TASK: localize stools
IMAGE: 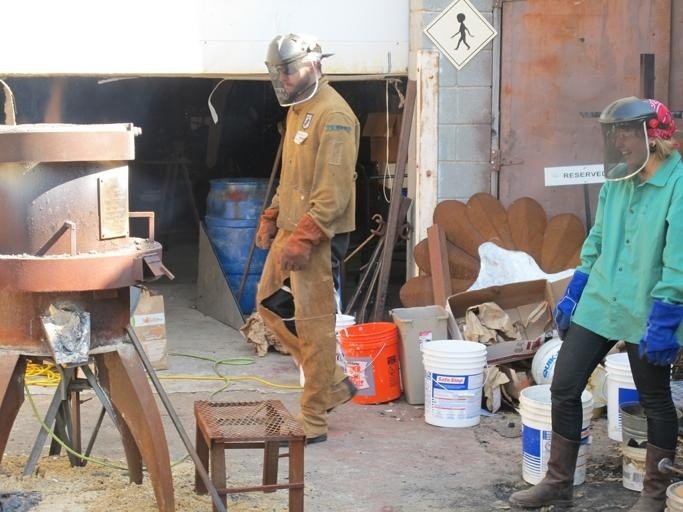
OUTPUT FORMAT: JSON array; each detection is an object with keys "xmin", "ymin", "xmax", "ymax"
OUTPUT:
[{"xmin": 193, "ymin": 399, "xmax": 306, "ymax": 512}]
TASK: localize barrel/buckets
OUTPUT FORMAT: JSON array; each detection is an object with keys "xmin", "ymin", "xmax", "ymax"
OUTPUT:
[
  {"xmin": 420, "ymin": 339, "xmax": 488, "ymax": 428},
  {"xmin": 299, "ymin": 314, "xmax": 357, "ymax": 389},
  {"xmin": 531, "ymin": 338, "xmax": 607, "ymax": 409},
  {"xmin": 206, "ymin": 179, "xmax": 270, "ymax": 314},
  {"xmin": 664, "ymin": 481, "xmax": 683, "ymax": 512},
  {"xmin": 339, "ymin": 321, "xmax": 400, "ymax": 404},
  {"xmin": 621, "ymin": 403, "xmax": 682, "ymax": 492},
  {"xmin": 604, "ymin": 352, "xmax": 641, "ymax": 440},
  {"xmin": 519, "ymin": 384, "xmax": 594, "ymax": 487}
]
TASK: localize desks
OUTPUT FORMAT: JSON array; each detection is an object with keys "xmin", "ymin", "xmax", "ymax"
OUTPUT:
[{"xmin": 136, "ymin": 159, "xmax": 201, "ymax": 226}]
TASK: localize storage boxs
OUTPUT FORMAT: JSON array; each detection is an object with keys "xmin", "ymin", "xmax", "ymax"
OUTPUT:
[
  {"xmin": 362, "ymin": 112, "xmax": 404, "ymax": 163},
  {"xmin": 129, "ymin": 285, "xmax": 167, "ymax": 370},
  {"xmin": 443, "ymin": 278, "xmax": 559, "ymax": 366}
]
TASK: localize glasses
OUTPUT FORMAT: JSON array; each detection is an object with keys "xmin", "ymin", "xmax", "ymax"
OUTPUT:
[
  {"xmin": 269, "ymin": 64, "xmax": 303, "ymax": 80},
  {"xmin": 606, "ymin": 129, "xmax": 635, "ymax": 144}
]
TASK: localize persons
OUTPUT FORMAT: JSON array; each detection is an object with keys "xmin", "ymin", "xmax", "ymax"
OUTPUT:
[
  {"xmin": 508, "ymin": 98, "xmax": 682, "ymax": 512},
  {"xmin": 254, "ymin": 33, "xmax": 363, "ymax": 447}
]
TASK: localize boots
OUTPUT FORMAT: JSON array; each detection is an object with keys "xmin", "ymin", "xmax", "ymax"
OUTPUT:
[
  {"xmin": 510, "ymin": 431, "xmax": 581, "ymax": 509},
  {"xmin": 628, "ymin": 442, "xmax": 676, "ymax": 512}
]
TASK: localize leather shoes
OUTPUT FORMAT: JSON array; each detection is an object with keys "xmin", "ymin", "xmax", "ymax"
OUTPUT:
[
  {"xmin": 328, "ymin": 379, "xmax": 357, "ymax": 413},
  {"xmin": 280, "ymin": 433, "xmax": 328, "ymax": 448}
]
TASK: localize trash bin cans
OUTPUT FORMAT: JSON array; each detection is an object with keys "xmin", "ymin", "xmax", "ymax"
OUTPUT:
[{"xmin": 389, "ymin": 304, "xmax": 449, "ymax": 405}]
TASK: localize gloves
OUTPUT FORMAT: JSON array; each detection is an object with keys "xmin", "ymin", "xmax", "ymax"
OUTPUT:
[
  {"xmin": 276, "ymin": 213, "xmax": 329, "ymax": 272},
  {"xmin": 553, "ymin": 270, "xmax": 590, "ymax": 341},
  {"xmin": 638, "ymin": 299, "xmax": 683, "ymax": 366},
  {"xmin": 254, "ymin": 209, "xmax": 277, "ymax": 250}
]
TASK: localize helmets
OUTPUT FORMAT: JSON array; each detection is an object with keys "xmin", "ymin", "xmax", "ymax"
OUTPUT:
[
  {"xmin": 599, "ymin": 96, "xmax": 676, "ymax": 139},
  {"xmin": 264, "ymin": 33, "xmax": 322, "ymax": 68}
]
